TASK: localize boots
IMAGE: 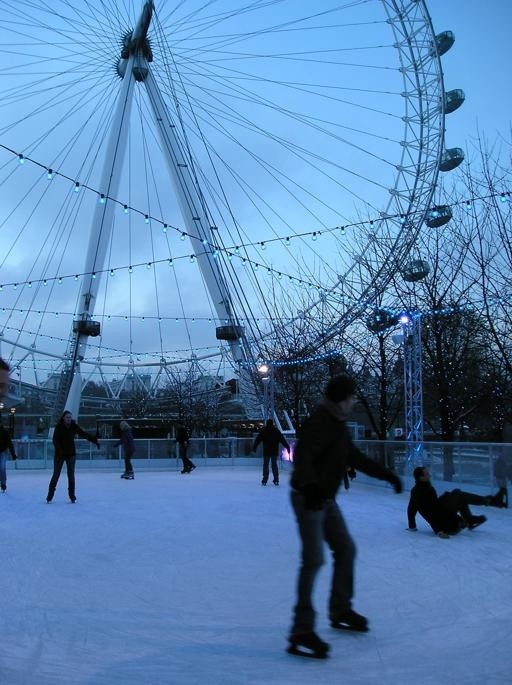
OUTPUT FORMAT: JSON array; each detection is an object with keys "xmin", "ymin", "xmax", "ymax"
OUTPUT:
[
  {"xmin": 287, "ymin": 619, "xmax": 332, "ymax": 653},
  {"xmin": 463, "ymin": 486, "xmax": 506, "ymax": 527},
  {"xmin": 329, "ymin": 601, "xmax": 370, "ymax": 627}
]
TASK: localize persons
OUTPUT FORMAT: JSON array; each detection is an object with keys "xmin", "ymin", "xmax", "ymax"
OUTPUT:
[
  {"xmin": 0, "ymin": 424, "xmax": 15, "ymax": 494},
  {"xmin": 344, "ymin": 468, "xmax": 358, "ymax": 491},
  {"xmin": 404, "ymin": 465, "xmax": 508, "ymax": 539},
  {"xmin": 111, "ymin": 420, "xmax": 136, "ymax": 479},
  {"xmin": 284, "ymin": 375, "xmax": 402, "ymax": 661},
  {"xmin": 252, "ymin": 418, "xmax": 291, "ymax": 486},
  {"xmin": 173, "ymin": 421, "xmax": 196, "ymax": 473},
  {"xmin": 44, "ymin": 409, "xmax": 99, "ymax": 504},
  {"xmin": 0, "ymin": 356, "xmax": 12, "ymax": 409}
]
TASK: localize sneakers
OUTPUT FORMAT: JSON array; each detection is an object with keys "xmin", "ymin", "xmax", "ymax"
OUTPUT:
[{"xmin": 1, "ymin": 461, "xmax": 279, "ymax": 501}]
